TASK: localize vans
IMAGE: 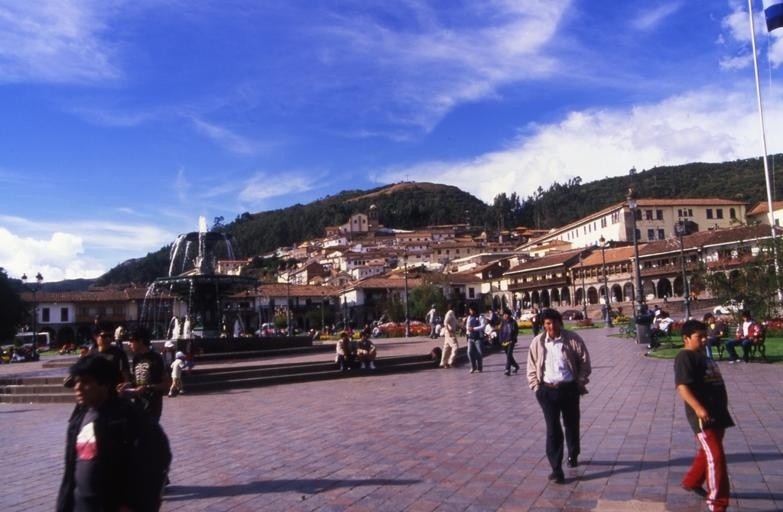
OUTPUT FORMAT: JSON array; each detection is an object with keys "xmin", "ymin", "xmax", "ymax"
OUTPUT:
[{"xmin": 254, "ymin": 323, "xmax": 287, "ymax": 338}]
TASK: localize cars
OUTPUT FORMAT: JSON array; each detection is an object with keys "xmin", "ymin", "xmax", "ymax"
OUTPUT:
[
  {"xmin": 761, "ymin": 314, "xmax": 782, "ymax": 330},
  {"xmin": 712, "ymin": 296, "xmax": 744, "ymax": 316},
  {"xmin": 560, "ymin": 310, "xmax": 583, "ymax": 322},
  {"xmin": 318, "ymin": 327, "xmax": 361, "ymax": 342},
  {"xmin": 371, "ymin": 318, "xmax": 429, "ymax": 339},
  {"xmin": 599, "ymin": 310, "xmax": 625, "ymax": 320},
  {"xmin": 518, "ymin": 314, "xmax": 538, "ymax": 328},
  {"xmin": 57, "ymin": 338, "xmax": 96, "ymax": 354}
]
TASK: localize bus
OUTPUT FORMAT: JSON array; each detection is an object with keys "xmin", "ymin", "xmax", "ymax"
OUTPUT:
[{"xmin": 12, "ymin": 332, "xmax": 50, "ymax": 353}]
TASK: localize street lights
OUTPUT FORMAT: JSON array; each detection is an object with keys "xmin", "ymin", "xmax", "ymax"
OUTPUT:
[
  {"xmin": 625, "ymin": 183, "xmax": 647, "ymax": 317},
  {"xmin": 598, "ymin": 233, "xmax": 612, "ymax": 326},
  {"xmin": 244, "ymin": 288, "xmax": 262, "ymax": 337},
  {"xmin": 21, "ymin": 271, "xmax": 43, "ymax": 359},
  {"xmin": 319, "ymin": 291, "xmax": 325, "ymax": 333},
  {"xmin": 487, "ymin": 269, "xmax": 494, "ymax": 312},
  {"xmin": 577, "ymin": 253, "xmax": 588, "ymax": 320},
  {"xmin": 341, "ymin": 284, "xmax": 349, "ymax": 332},
  {"xmin": 673, "ymin": 217, "xmax": 693, "ymax": 322},
  {"xmin": 402, "ymin": 263, "xmax": 409, "ymax": 337}
]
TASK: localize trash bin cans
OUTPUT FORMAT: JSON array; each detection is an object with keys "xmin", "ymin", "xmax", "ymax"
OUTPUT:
[{"xmin": 635, "ymin": 315, "xmax": 651, "ymax": 344}]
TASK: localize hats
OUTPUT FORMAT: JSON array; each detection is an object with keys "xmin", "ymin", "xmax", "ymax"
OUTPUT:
[{"xmin": 176, "ymin": 351, "xmax": 185, "ymax": 358}]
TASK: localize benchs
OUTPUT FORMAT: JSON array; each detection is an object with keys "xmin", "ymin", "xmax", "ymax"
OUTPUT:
[
  {"xmin": 710, "ymin": 325, "xmax": 768, "ymax": 362},
  {"xmin": 652, "ymin": 323, "xmax": 675, "ymax": 351}
]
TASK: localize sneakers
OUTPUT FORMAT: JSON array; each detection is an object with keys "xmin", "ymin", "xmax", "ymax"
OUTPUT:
[
  {"xmin": 504, "ymin": 369, "xmax": 510, "ymax": 376},
  {"xmin": 513, "ymin": 365, "xmax": 520, "ymax": 373}
]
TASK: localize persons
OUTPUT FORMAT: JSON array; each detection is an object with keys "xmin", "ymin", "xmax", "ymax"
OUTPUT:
[
  {"xmin": 333, "ymin": 332, "xmax": 353, "ymax": 372},
  {"xmin": 327, "ymin": 322, "xmax": 336, "ymax": 335},
  {"xmin": 645, "ymin": 304, "xmax": 673, "ymax": 348},
  {"xmin": 372, "ymin": 323, "xmax": 382, "ymax": 337},
  {"xmin": 0, "ymin": 345, "xmax": 40, "ymax": 364},
  {"xmin": 725, "ymin": 309, "xmax": 762, "ymax": 363},
  {"xmin": 53, "ymin": 322, "xmax": 193, "ymax": 512},
  {"xmin": 423, "ymin": 300, "xmax": 521, "ymax": 376},
  {"xmin": 354, "ymin": 332, "xmax": 376, "ymax": 369},
  {"xmin": 701, "ymin": 313, "xmax": 726, "ymax": 360},
  {"xmin": 525, "ymin": 308, "xmax": 591, "ymax": 482},
  {"xmin": 672, "ymin": 319, "xmax": 736, "ymax": 511},
  {"xmin": 528, "ymin": 308, "xmax": 540, "ymax": 336},
  {"xmin": 361, "ymin": 323, "xmax": 371, "ymax": 338}
]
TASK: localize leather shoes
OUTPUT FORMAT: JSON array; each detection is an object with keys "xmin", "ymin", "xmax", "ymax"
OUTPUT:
[
  {"xmin": 682, "ymin": 484, "xmax": 707, "ymax": 498},
  {"xmin": 548, "ymin": 473, "xmax": 564, "ymax": 484},
  {"xmin": 569, "ymin": 457, "xmax": 577, "ymax": 467}
]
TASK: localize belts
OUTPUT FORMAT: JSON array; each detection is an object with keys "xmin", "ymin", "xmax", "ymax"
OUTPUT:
[{"xmin": 543, "ymin": 382, "xmax": 561, "ymax": 388}]
